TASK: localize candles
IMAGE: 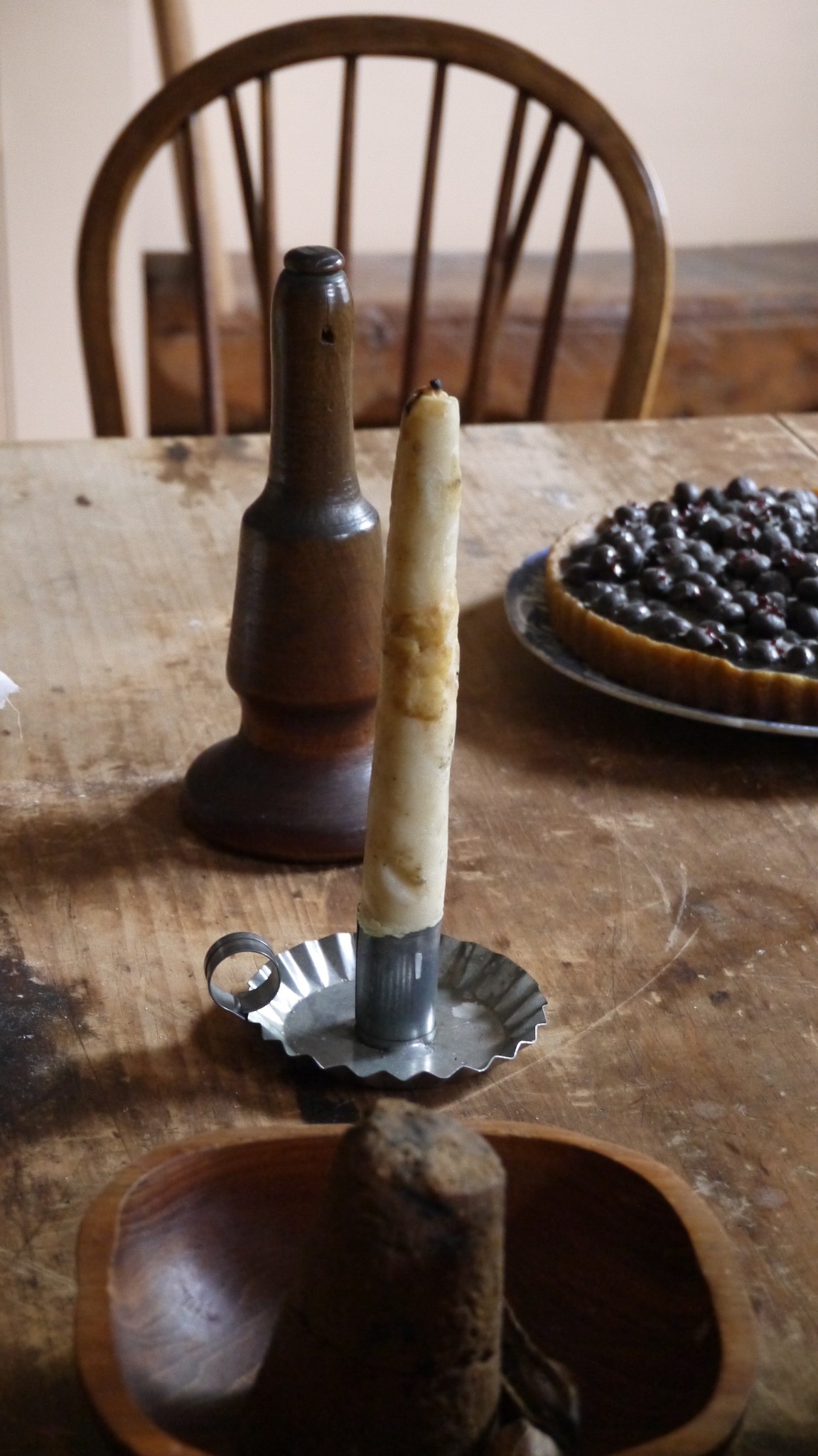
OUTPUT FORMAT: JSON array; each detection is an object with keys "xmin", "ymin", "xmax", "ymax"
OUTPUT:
[{"xmin": 356, "ymin": 378, "xmax": 469, "ymax": 940}]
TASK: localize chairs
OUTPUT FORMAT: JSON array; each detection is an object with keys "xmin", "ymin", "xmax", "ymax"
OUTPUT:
[{"xmin": 76, "ymin": 16, "xmax": 679, "ymax": 440}]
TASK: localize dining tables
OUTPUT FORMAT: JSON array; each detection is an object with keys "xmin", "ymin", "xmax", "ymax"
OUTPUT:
[{"xmin": 0, "ymin": 415, "xmax": 818, "ymax": 1456}]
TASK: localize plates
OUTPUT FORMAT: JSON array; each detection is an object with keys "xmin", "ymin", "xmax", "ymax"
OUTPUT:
[{"xmin": 504, "ymin": 547, "xmax": 818, "ymax": 737}]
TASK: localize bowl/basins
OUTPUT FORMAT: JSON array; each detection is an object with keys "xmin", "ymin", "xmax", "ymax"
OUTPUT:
[{"xmin": 69, "ymin": 1124, "xmax": 758, "ymax": 1456}]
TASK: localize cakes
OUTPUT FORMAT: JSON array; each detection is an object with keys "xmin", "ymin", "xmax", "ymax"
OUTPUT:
[{"xmin": 543, "ymin": 476, "xmax": 817, "ymax": 726}]
